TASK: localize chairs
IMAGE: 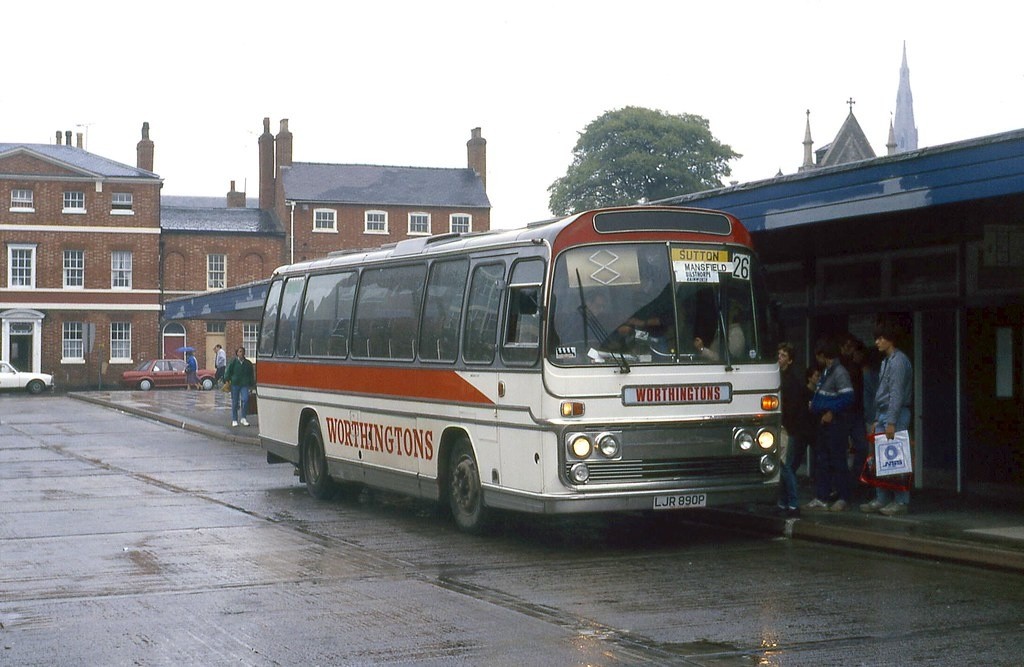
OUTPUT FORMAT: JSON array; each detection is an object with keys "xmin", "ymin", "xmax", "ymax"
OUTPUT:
[
  {"xmin": 411, "ymin": 339, "xmax": 438, "ymax": 358},
  {"xmin": 436, "ymin": 337, "xmax": 458, "ymax": 360},
  {"xmin": 310, "ymin": 338, "xmax": 328, "ymax": 355},
  {"xmin": 292, "ymin": 336, "xmax": 311, "ymax": 355},
  {"xmin": 327, "ymin": 335, "xmax": 347, "ymax": 356},
  {"xmin": 346, "ymin": 337, "xmax": 367, "ymax": 357},
  {"xmin": 388, "ymin": 338, "xmax": 413, "ymax": 357},
  {"xmin": 366, "ymin": 338, "xmax": 389, "ymax": 357}
]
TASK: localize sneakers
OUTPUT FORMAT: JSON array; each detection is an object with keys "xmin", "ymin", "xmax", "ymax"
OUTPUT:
[
  {"xmin": 828, "ymin": 500, "xmax": 852, "ymax": 512},
  {"xmin": 878, "ymin": 502, "xmax": 908, "ymax": 516},
  {"xmin": 860, "ymin": 498, "xmax": 884, "ymax": 512},
  {"xmin": 800, "ymin": 497, "xmax": 829, "ymax": 511}
]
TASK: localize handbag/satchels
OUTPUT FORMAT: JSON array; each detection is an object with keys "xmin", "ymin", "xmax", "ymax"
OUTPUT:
[
  {"xmin": 222, "ymin": 382, "xmax": 231, "ymax": 393},
  {"xmin": 246, "ymin": 389, "xmax": 258, "ymax": 415},
  {"xmin": 860, "ymin": 430, "xmax": 915, "ymax": 493},
  {"xmin": 185, "ymin": 366, "xmax": 192, "ymax": 373}
]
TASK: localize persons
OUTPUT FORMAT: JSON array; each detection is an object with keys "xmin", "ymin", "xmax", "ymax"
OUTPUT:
[
  {"xmin": 184, "ymin": 351, "xmax": 204, "ymax": 390},
  {"xmin": 213, "ymin": 344, "xmax": 228, "ymax": 389},
  {"xmin": 773, "ymin": 331, "xmax": 876, "ymax": 511},
  {"xmin": 224, "ymin": 346, "xmax": 255, "ymax": 426},
  {"xmin": 857, "ymin": 322, "xmax": 913, "ymax": 516},
  {"xmin": 556, "ymin": 263, "xmax": 745, "ymax": 361}
]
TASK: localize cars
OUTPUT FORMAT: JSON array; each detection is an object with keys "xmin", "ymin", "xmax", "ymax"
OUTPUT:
[
  {"xmin": 122, "ymin": 359, "xmax": 219, "ymax": 391},
  {"xmin": 0, "ymin": 360, "xmax": 54, "ymax": 394}
]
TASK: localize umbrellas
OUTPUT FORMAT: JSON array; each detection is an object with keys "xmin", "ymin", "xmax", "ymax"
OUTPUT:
[{"xmin": 176, "ymin": 346, "xmax": 197, "ymax": 353}]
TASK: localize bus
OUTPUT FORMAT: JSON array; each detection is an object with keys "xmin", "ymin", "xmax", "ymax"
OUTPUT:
[{"xmin": 254, "ymin": 203, "xmax": 784, "ymax": 537}]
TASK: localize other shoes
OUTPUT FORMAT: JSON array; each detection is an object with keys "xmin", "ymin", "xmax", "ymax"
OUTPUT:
[
  {"xmin": 784, "ymin": 506, "xmax": 801, "ymax": 518},
  {"xmin": 241, "ymin": 418, "xmax": 251, "ymax": 426},
  {"xmin": 232, "ymin": 421, "xmax": 238, "ymax": 427}
]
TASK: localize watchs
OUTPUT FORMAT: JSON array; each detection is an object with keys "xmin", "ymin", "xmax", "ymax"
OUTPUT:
[{"xmin": 699, "ymin": 347, "xmax": 704, "ymax": 352}]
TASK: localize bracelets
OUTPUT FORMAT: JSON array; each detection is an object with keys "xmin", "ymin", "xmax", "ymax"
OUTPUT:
[{"xmin": 644, "ymin": 319, "xmax": 649, "ymax": 328}]
